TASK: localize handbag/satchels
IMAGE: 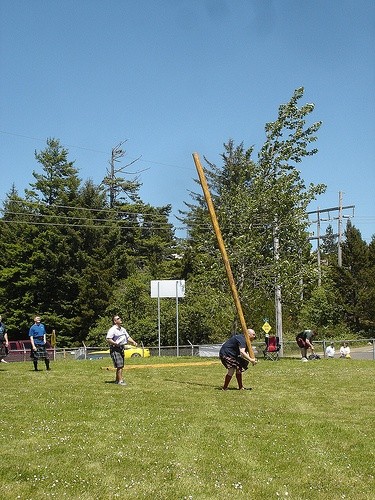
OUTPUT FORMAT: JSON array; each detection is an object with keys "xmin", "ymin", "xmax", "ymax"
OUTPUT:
[{"xmin": 345, "ymin": 354, "xmax": 351, "ymax": 359}]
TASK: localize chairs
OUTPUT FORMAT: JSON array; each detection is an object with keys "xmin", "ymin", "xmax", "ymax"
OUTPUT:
[{"xmin": 262, "ymin": 336, "xmax": 281, "ymax": 361}]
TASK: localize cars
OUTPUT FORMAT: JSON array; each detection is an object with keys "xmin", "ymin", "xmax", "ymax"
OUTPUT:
[{"xmin": 88, "ymin": 345, "xmax": 151, "ymax": 360}]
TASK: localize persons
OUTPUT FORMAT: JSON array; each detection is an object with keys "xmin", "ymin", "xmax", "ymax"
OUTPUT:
[
  {"xmin": 0, "ymin": 315, "xmax": 9, "ymax": 347},
  {"xmin": 326, "ymin": 342, "xmax": 335, "ymax": 358},
  {"xmin": 296, "ymin": 329, "xmax": 318, "ymax": 361},
  {"xmin": 106, "ymin": 315, "xmax": 137, "ymax": 385},
  {"xmin": 29, "ymin": 315, "xmax": 51, "ymax": 371},
  {"xmin": 340, "ymin": 342, "xmax": 350, "ymax": 358},
  {"xmin": 219, "ymin": 329, "xmax": 257, "ymax": 390}
]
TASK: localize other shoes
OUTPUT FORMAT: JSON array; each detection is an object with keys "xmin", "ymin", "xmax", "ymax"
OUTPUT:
[
  {"xmin": 1, "ymin": 358, "xmax": 8, "ymax": 364},
  {"xmin": 115, "ymin": 379, "xmax": 127, "ymax": 386},
  {"xmin": 237, "ymin": 387, "xmax": 246, "ymax": 391},
  {"xmin": 222, "ymin": 387, "xmax": 230, "ymax": 392},
  {"xmin": 300, "ymin": 357, "xmax": 309, "ymax": 362}
]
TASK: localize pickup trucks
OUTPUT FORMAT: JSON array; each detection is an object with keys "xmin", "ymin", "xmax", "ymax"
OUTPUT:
[{"xmin": 3, "ymin": 339, "xmax": 54, "ymax": 361}]
